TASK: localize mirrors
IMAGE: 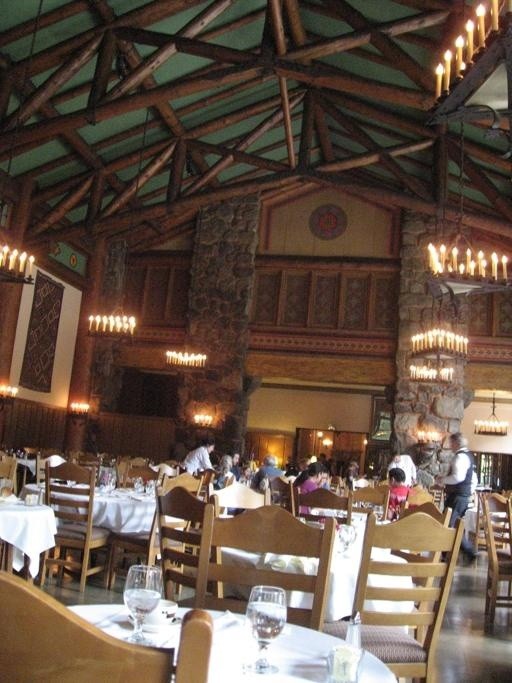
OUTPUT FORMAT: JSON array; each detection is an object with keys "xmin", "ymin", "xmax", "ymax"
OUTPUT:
[{"xmin": 368, "ymin": 390, "xmax": 395, "ymax": 448}]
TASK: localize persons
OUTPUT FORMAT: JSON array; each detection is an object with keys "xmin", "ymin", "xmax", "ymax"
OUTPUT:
[
  {"xmin": 239, "ymin": 464, "xmax": 251, "ymax": 488},
  {"xmin": 386, "ymin": 448, "xmax": 417, "ymax": 487},
  {"xmin": 293, "ymin": 463, "xmax": 323, "ymax": 513},
  {"xmin": 285, "ymin": 458, "xmax": 312, "ymax": 482},
  {"xmin": 386, "ymin": 469, "xmax": 417, "ymax": 520},
  {"xmin": 182, "ymin": 437, "xmax": 215, "ymax": 476},
  {"xmin": 251, "ymin": 456, "xmax": 282, "ymax": 489},
  {"xmin": 350, "ymin": 457, "xmax": 359, "ymax": 475},
  {"xmin": 435, "ymin": 432, "xmax": 481, "ymax": 567},
  {"xmin": 320, "ymin": 454, "xmax": 332, "ymax": 477},
  {"xmin": 213, "ymin": 455, "xmax": 236, "ymax": 490},
  {"xmin": 346, "ymin": 464, "xmax": 354, "ymax": 490},
  {"xmin": 230, "ymin": 452, "xmax": 241, "ymax": 481}
]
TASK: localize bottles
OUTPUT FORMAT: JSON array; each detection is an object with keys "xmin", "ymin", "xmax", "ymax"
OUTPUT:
[
  {"xmin": 39, "ymin": 489, "xmax": 45, "ymax": 505},
  {"xmin": 344, "ymin": 619, "xmax": 364, "ymax": 682}
]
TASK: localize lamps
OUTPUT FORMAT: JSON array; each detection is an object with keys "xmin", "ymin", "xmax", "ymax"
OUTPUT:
[
  {"xmin": 421, "ymin": 1, "xmax": 510, "ymax": 119},
  {"xmin": 86, "ymin": 106, "xmax": 150, "ymax": 335},
  {"xmin": 192, "ymin": 411, "xmax": 214, "ymax": 426},
  {"xmin": 417, "ymin": 430, "xmax": 440, "ymax": 442},
  {"xmin": 69, "ymin": 401, "xmax": 91, "ymax": 415},
  {"xmin": 411, "ymin": 119, "xmax": 470, "ymax": 360},
  {"xmin": 408, "ymin": 364, "xmax": 456, "ymax": 384},
  {"xmin": 474, "ymin": 392, "xmax": 508, "ymax": 437},
  {"xmin": 422, "ymin": 118, "xmax": 512, "ymax": 296},
  {"xmin": 0, "ymin": 386, "xmax": 18, "ymax": 402},
  {"xmin": 165, "ymin": 207, "xmax": 208, "ymax": 368},
  {"xmin": 0, "ymin": 0, "xmax": 42, "ymax": 285}
]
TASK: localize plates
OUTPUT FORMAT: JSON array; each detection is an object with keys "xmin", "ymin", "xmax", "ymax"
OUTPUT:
[{"xmin": 129, "ymin": 618, "xmax": 179, "ymax": 631}]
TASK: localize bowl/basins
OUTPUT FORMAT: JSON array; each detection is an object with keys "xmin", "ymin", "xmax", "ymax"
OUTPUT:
[{"xmin": 131, "ymin": 601, "xmax": 178, "ymax": 624}]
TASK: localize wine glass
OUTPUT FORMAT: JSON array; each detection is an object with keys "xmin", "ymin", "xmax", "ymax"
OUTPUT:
[
  {"xmin": 247, "ymin": 585, "xmax": 287, "ymax": 674},
  {"xmin": 124, "ymin": 480, "xmax": 136, "ymax": 501},
  {"xmin": 124, "ymin": 565, "xmax": 162, "ymax": 646},
  {"xmin": 339, "ymin": 525, "xmax": 356, "ymax": 556}
]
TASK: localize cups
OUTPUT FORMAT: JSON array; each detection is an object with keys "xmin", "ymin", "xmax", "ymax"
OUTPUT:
[
  {"xmin": 326, "ymin": 647, "xmax": 364, "ymax": 683},
  {"xmin": 24, "ymin": 494, "xmax": 38, "ymax": 505}
]
